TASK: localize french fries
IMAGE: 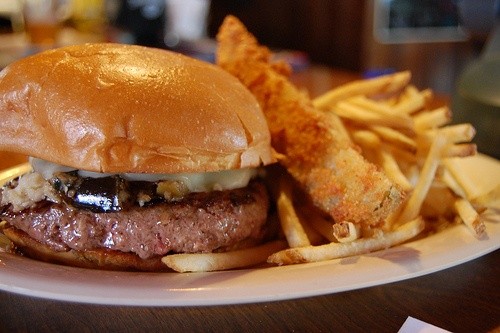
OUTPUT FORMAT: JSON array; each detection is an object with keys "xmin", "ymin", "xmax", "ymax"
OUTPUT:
[{"xmin": 161, "ymin": 69, "xmax": 500, "ymax": 273}]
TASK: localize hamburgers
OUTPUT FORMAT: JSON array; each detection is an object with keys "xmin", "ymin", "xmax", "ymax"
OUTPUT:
[{"xmin": 0, "ymin": 42, "xmax": 284, "ymax": 270}]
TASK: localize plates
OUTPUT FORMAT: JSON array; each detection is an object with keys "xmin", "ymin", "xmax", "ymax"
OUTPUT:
[{"xmin": 0, "ymin": 64, "xmax": 500, "ymax": 306}]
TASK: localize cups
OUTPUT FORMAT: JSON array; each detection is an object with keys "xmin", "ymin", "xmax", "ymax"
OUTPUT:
[
  {"xmin": 22, "ymin": 0, "xmax": 62, "ymax": 52},
  {"xmin": 164, "ymin": 0, "xmax": 208, "ymax": 43}
]
